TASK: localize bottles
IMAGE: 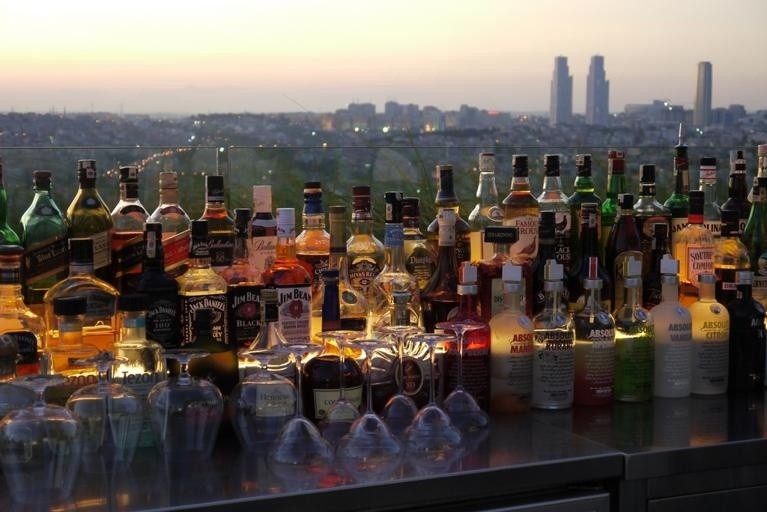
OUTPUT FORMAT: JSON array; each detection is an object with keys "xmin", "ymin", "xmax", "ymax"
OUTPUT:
[{"xmin": 0, "ymin": 145, "xmax": 767, "ymax": 506}]
